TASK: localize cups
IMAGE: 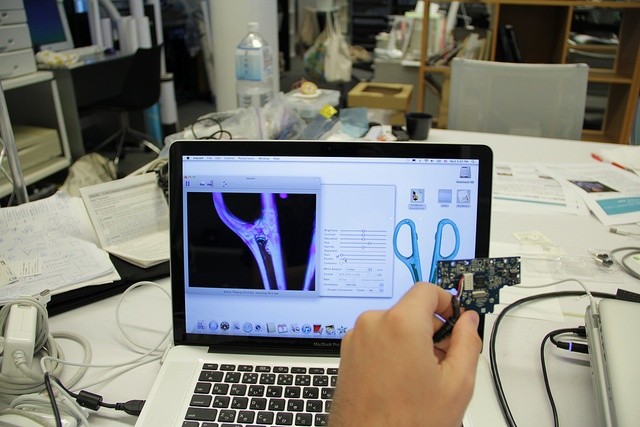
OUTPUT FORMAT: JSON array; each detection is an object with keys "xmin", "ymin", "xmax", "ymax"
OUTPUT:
[{"xmin": 405, "ymin": 112, "xmax": 433, "ymax": 140}]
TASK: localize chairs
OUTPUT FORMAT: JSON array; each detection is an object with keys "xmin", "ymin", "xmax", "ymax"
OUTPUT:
[
  {"xmin": 447, "ymin": 56, "xmax": 590, "ymax": 141},
  {"xmin": 72, "ymin": 41, "xmax": 160, "ymax": 176}
]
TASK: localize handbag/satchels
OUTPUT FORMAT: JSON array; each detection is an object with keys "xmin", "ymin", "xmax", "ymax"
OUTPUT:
[{"xmin": 303, "ymin": 31, "xmax": 353, "ymax": 82}]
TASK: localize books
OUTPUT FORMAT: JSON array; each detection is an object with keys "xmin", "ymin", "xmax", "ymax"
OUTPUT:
[{"xmin": 426, "ymin": 30, "xmax": 491, "ymax": 68}]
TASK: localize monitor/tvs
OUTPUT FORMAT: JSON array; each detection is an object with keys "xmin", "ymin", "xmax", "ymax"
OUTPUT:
[{"xmin": 23, "ymin": 1, "xmax": 76, "ymax": 53}]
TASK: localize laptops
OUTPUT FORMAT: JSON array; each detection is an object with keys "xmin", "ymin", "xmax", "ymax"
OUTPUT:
[
  {"xmin": 132, "ymin": 139, "xmax": 494, "ymax": 427},
  {"xmin": 583, "ymin": 298, "xmax": 639, "ymax": 427}
]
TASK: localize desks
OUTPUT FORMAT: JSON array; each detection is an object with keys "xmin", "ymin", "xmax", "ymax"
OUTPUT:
[{"xmin": 0, "ymin": 130, "xmax": 636, "ymax": 427}]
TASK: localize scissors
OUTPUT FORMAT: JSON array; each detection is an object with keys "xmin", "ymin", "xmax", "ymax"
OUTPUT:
[{"xmin": 392, "ymin": 218, "xmax": 460, "ymax": 284}]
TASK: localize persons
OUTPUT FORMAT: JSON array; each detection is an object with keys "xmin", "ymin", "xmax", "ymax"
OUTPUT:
[{"xmin": 324, "ymin": 280, "xmax": 483, "ymax": 426}]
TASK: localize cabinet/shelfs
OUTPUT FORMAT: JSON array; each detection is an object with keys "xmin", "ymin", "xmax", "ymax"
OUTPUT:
[
  {"xmin": 1, "ymin": 71, "xmax": 74, "ymax": 197},
  {"xmin": 419, "ymin": 0, "xmax": 640, "ymax": 144},
  {"xmin": 0, "ymin": 0, "xmax": 37, "ymax": 79}
]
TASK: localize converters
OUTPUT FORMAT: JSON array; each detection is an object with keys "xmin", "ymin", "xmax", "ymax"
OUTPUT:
[{"xmin": 1, "ymin": 303, "xmax": 39, "ymax": 379}]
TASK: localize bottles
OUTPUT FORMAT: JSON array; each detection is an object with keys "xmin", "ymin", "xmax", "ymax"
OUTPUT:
[{"xmin": 234, "ymin": 20, "xmax": 274, "ymax": 108}]
